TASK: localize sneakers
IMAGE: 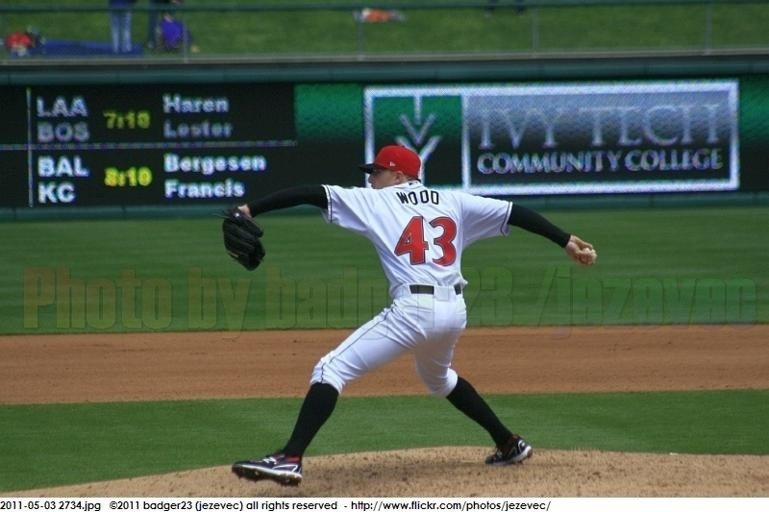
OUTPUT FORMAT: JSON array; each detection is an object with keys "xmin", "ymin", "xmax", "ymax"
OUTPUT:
[
  {"xmin": 232, "ymin": 451, "xmax": 302, "ymax": 485},
  {"xmin": 485, "ymin": 434, "xmax": 532, "ymax": 465}
]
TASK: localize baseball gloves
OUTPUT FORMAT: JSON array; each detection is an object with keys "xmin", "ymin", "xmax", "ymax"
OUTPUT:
[{"xmin": 223, "ymin": 207, "xmax": 264, "ymax": 269}]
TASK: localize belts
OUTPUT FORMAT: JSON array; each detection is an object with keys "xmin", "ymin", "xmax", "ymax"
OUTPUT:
[{"xmin": 410, "ymin": 284, "xmax": 461, "ymax": 294}]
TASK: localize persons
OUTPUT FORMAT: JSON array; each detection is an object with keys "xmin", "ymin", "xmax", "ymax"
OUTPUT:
[
  {"xmin": 222, "ymin": 143, "xmax": 597, "ymax": 486},
  {"xmin": 109, "ymin": 0, "xmax": 133, "ymax": 54},
  {"xmin": 148, "ymin": 0, "xmax": 179, "ymax": 52}
]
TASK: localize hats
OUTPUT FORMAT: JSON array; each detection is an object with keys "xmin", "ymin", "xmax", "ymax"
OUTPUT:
[{"xmin": 359, "ymin": 145, "xmax": 420, "ymax": 176}]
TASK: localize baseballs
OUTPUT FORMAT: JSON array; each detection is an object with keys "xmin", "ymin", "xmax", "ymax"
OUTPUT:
[{"xmin": 581, "ymin": 247, "xmax": 596, "ymax": 263}]
